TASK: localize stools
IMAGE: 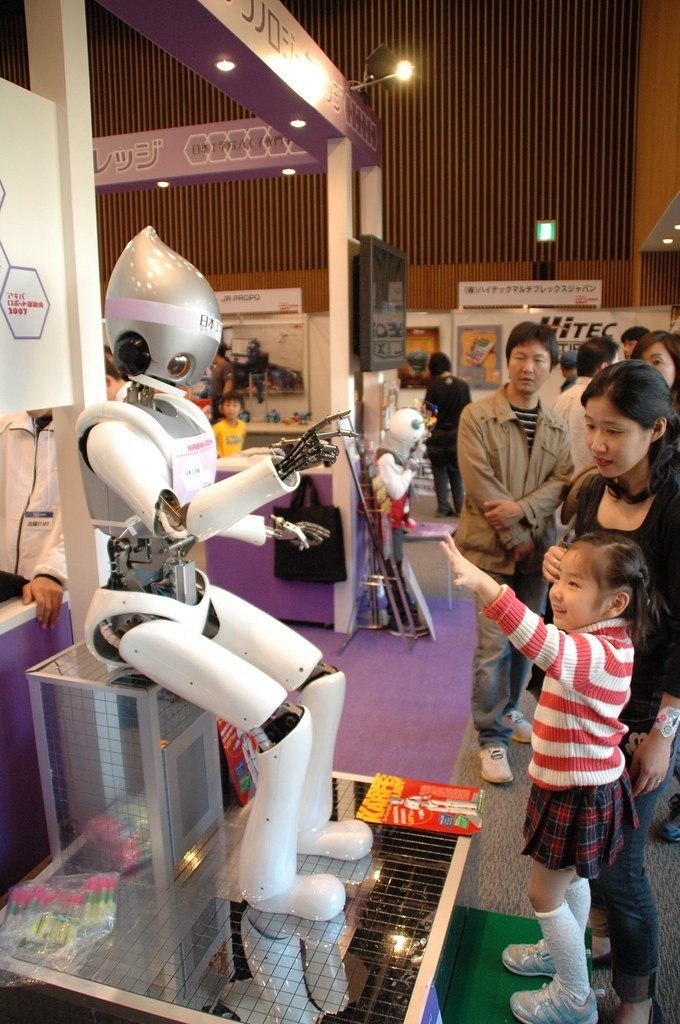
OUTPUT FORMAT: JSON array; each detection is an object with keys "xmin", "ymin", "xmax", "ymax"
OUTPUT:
[{"xmin": 403, "ymin": 523, "xmax": 459, "ymax": 611}]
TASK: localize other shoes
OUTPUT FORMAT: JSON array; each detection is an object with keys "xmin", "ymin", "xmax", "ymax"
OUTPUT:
[
  {"xmin": 587, "ymin": 949, "xmax": 612, "ymax": 969},
  {"xmin": 650, "ymin": 998, "xmax": 662, "ymax": 1024},
  {"xmin": 438, "ymin": 508, "xmax": 454, "ymax": 518}
]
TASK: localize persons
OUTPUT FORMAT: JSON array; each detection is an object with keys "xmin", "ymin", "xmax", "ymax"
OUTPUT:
[
  {"xmin": 104, "ymin": 344, "xmax": 156, "ymax": 411},
  {"xmin": 439, "ymin": 531, "xmax": 671, "ymax": 1024},
  {"xmin": 209, "ymin": 345, "xmax": 247, "ymax": 459},
  {"xmin": 0, "ymin": 408, "xmax": 68, "ymax": 630},
  {"xmin": 440, "ymin": 320, "xmax": 680, "ymax": 842},
  {"xmin": 521, "ymin": 359, "xmax": 680, "ymax": 1024},
  {"xmin": 422, "ymin": 351, "xmax": 472, "ymax": 519}
]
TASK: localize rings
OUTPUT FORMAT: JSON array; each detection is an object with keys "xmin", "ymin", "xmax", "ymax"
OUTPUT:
[
  {"xmin": 656, "ymin": 780, "xmax": 662, "ymax": 783},
  {"xmin": 552, "ymin": 559, "xmax": 558, "ymax": 566}
]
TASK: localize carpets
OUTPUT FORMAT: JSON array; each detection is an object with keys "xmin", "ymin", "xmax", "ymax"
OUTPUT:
[{"xmin": 259, "ymin": 597, "xmax": 486, "ymax": 786}]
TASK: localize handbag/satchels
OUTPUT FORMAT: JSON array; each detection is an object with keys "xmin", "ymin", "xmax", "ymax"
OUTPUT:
[{"xmin": 272, "ymin": 474, "xmax": 348, "ymax": 583}]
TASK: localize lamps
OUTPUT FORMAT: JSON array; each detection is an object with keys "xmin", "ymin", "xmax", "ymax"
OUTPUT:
[{"xmin": 349, "ymin": 60, "xmax": 419, "ymax": 93}]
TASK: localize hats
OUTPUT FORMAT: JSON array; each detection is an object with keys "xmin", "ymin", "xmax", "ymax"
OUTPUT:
[{"xmin": 560, "ymin": 351, "xmax": 578, "ymax": 369}]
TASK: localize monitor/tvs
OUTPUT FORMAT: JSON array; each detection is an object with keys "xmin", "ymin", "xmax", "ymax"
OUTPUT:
[{"xmin": 351, "ymin": 234, "xmax": 407, "ymax": 372}]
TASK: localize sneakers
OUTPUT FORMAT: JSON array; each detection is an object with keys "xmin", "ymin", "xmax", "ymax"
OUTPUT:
[
  {"xmin": 479, "ymin": 748, "xmax": 513, "ymax": 783},
  {"xmin": 510, "ymin": 982, "xmax": 605, "ymax": 1024},
  {"xmin": 502, "ymin": 710, "xmax": 533, "ymax": 742},
  {"xmin": 658, "ymin": 805, "xmax": 680, "ymax": 842},
  {"xmin": 502, "ymin": 939, "xmax": 591, "ymax": 976}
]
